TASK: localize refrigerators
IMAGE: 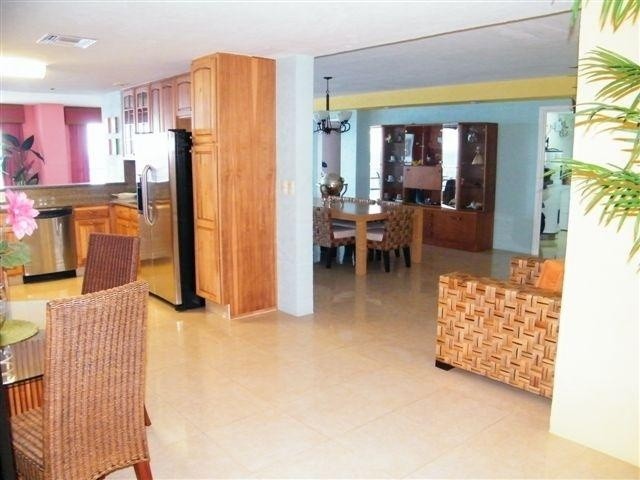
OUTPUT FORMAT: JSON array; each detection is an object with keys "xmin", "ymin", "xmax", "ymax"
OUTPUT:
[{"xmin": 132, "ymin": 129, "xmax": 205, "ymax": 311}]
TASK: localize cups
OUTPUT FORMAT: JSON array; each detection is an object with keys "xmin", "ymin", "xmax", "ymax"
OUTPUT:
[{"xmin": 384, "ymin": 156, "xmax": 404, "ymax": 202}]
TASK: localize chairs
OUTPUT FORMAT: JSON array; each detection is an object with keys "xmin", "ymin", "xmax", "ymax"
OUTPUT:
[
  {"xmin": 9, "ymin": 232, "xmax": 155, "ymax": 480},
  {"xmin": 314, "ymin": 195, "xmax": 414, "ymax": 272},
  {"xmin": 434, "ymin": 256, "xmax": 565, "ymax": 399}
]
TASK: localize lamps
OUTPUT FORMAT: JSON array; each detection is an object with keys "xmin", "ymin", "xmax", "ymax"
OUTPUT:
[{"xmin": 311, "ymin": 77, "xmax": 351, "ymax": 135}]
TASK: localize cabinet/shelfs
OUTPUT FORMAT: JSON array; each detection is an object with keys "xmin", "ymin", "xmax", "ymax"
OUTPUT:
[
  {"xmin": 109, "ymin": 204, "xmax": 139, "ymax": 239},
  {"xmin": 174, "ymin": 72, "xmax": 192, "ymax": 118},
  {"xmin": 120, "ymin": 84, "xmax": 151, "ymax": 162},
  {"xmin": 380, "ymin": 121, "xmax": 499, "ymax": 252},
  {"xmin": 68, "ymin": 203, "xmax": 112, "ymax": 269},
  {"xmin": 152, "ymin": 78, "xmax": 176, "ymax": 133},
  {"xmin": 189, "ymin": 53, "xmax": 276, "ymax": 318}
]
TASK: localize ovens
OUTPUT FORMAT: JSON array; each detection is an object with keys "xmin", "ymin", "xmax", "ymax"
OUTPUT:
[{"xmin": 17, "ymin": 205, "xmax": 76, "ymax": 284}]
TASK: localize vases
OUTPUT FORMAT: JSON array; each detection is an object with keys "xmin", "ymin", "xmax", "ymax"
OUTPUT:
[{"xmin": 0, "ymin": 276, "xmax": 11, "ymax": 327}]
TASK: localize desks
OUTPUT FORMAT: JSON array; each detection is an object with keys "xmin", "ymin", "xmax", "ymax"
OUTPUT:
[{"xmin": 314, "ymin": 199, "xmax": 424, "ymax": 275}]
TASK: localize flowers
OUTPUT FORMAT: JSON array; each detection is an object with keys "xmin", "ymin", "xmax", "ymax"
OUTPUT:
[{"xmin": 1, "ymin": 184, "xmax": 42, "ymax": 285}]
